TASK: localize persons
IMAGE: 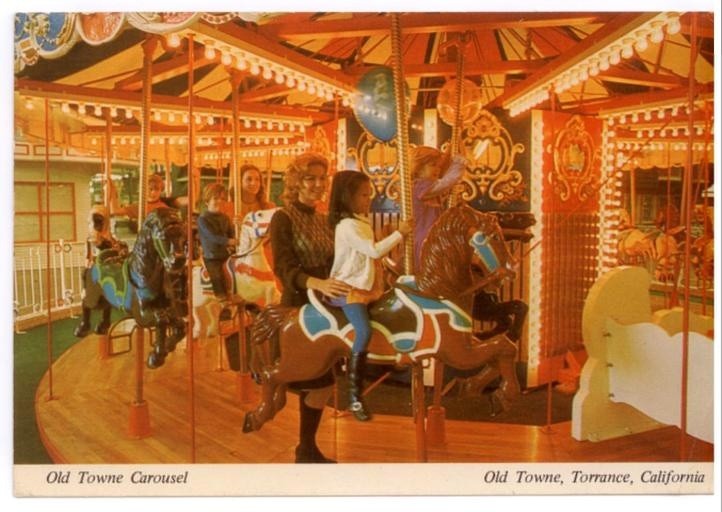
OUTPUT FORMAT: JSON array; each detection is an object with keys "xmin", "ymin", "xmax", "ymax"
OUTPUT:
[
  {"xmin": 73, "ymin": 206, "xmax": 189, "ymax": 369},
  {"xmin": 239, "ymin": 200, "xmax": 521, "ymax": 437},
  {"xmin": 261, "ymin": 149, "xmax": 350, "ymax": 462},
  {"xmin": 186, "ymin": 202, "xmax": 284, "ymax": 344},
  {"xmin": 195, "ymin": 181, "xmax": 235, "ymax": 263},
  {"xmin": 225, "ymin": 164, "xmax": 283, "ymax": 227},
  {"xmin": 616, "ymin": 196, "xmax": 714, "ymax": 283},
  {"xmin": 324, "ymin": 168, "xmax": 415, "ymax": 422},
  {"xmin": 107, "ymin": 171, "xmax": 190, "ymax": 219},
  {"xmin": 409, "ymin": 144, "xmax": 470, "ymax": 268},
  {"xmin": 468, "ymin": 286, "xmax": 526, "ymax": 342}
]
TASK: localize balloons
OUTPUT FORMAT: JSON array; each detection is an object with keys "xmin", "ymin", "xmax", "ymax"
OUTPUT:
[
  {"xmin": 351, "ymin": 64, "xmax": 410, "ymax": 146},
  {"xmin": 435, "ymin": 78, "xmax": 478, "ymax": 129}
]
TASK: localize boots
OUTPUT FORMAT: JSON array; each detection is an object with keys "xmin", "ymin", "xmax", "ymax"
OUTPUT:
[
  {"xmin": 294, "ymin": 398, "xmax": 339, "ymax": 463},
  {"xmin": 346, "ymin": 351, "xmax": 371, "ymax": 421}
]
